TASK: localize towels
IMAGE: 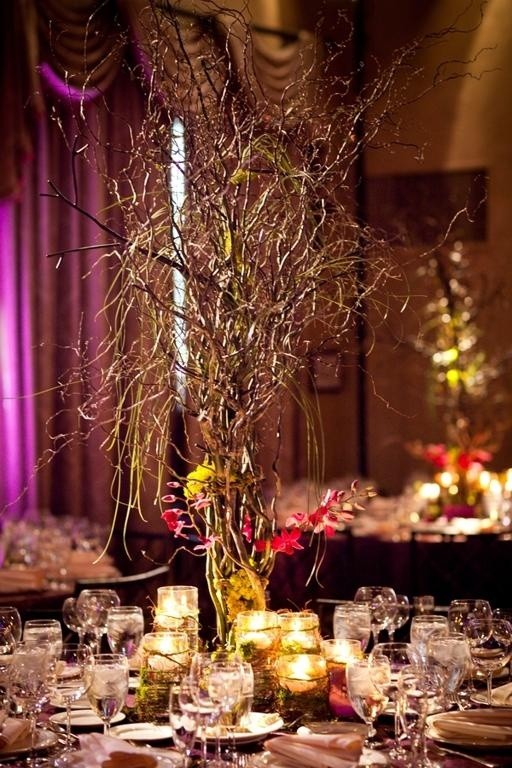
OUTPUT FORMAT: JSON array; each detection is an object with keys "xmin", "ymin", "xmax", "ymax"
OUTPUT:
[
  {"xmin": 263, "ymin": 731, "xmax": 364, "ymax": 768},
  {"xmin": 77, "ymin": 731, "xmax": 158, "ymax": 767},
  {"xmin": 431, "ymin": 706, "xmax": 512, "ymax": 748},
  {"xmin": 0, "ymin": 716, "xmax": 33, "ymax": 746}
]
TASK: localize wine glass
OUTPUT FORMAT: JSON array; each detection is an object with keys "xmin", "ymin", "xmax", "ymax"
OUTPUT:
[{"xmin": 0, "ymin": 581, "xmax": 512, "ymax": 768}]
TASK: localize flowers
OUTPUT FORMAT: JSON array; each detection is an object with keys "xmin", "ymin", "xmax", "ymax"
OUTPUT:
[
  {"xmin": 395, "ymin": 228, "xmax": 511, "ymax": 511},
  {"xmin": 0, "ymin": 0, "xmax": 505, "ymax": 614}
]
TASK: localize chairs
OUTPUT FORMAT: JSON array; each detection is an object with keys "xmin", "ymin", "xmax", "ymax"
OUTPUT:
[{"xmin": 73, "ymin": 565, "xmax": 174, "ymax": 629}]
